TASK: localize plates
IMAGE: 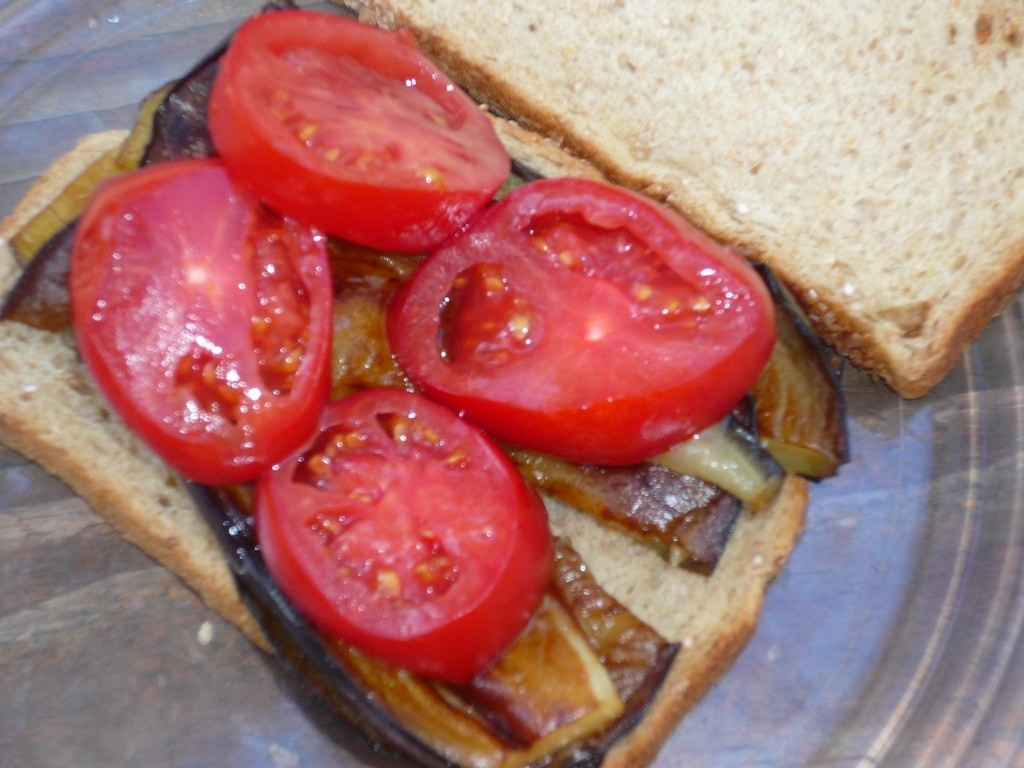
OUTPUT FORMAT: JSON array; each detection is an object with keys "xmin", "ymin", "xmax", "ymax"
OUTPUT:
[{"xmin": 0, "ymin": 0, "xmax": 1024, "ymax": 768}]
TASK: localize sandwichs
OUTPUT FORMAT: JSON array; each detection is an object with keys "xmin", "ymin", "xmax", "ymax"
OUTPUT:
[{"xmin": 0, "ymin": 0, "xmax": 1024, "ymax": 767}]
